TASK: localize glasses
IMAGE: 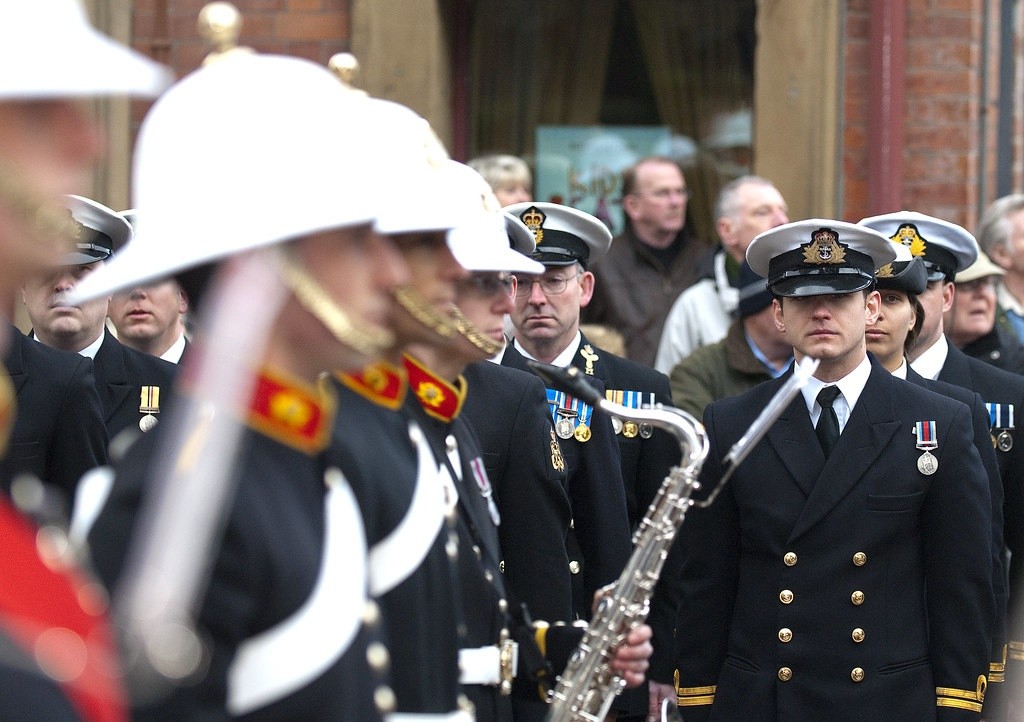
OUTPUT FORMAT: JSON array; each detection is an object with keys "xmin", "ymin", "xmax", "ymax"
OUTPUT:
[
  {"xmin": 634, "ymin": 188, "xmax": 694, "ymax": 201},
  {"xmin": 510, "ymin": 271, "xmax": 583, "ymax": 297}
]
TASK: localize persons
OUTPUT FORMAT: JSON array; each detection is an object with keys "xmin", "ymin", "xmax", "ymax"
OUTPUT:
[
  {"xmin": 495, "ymin": 203, "xmax": 675, "ymax": 722},
  {"xmin": 658, "ymin": 173, "xmax": 1024, "ymax": 722},
  {"xmin": 0, "ymin": 0, "xmax": 194, "ymax": 722},
  {"xmin": 328, "ymin": 94, "xmax": 652, "ymax": 722},
  {"xmin": 673, "ymin": 219, "xmax": 995, "ymax": 722},
  {"xmin": 580, "ymin": 156, "xmax": 723, "ymax": 371},
  {"xmin": 63, "ymin": 55, "xmax": 409, "ymax": 721}
]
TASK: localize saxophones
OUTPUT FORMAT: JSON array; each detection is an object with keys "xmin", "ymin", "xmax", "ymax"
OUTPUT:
[{"xmin": 525, "ymin": 357, "xmax": 817, "ymax": 722}]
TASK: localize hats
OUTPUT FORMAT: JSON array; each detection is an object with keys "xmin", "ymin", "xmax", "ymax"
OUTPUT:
[
  {"xmin": 739, "ymin": 259, "xmax": 779, "ymax": 319},
  {"xmin": 857, "ymin": 211, "xmax": 978, "ymax": 281},
  {"xmin": 502, "ymin": 201, "xmax": 613, "ymax": 269},
  {"xmin": 40, "ymin": 191, "xmax": 129, "ymax": 266},
  {"xmin": 873, "ymin": 239, "xmax": 928, "ymax": 295},
  {"xmin": 955, "ymin": 249, "xmax": 1007, "ymax": 284},
  {"xmin": 745, "ymin": 218, "xmax": 898, "ymax": 298}
]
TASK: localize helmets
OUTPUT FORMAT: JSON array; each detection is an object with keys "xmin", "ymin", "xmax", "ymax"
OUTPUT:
[
  {"xmin": 3, "ymin": 6, "xmax": 175, "ymax": 100},
  {"xmin": 61, "ymin": 2, "xmax": 377, "ymax": 310},
  {"xmin": 438, "ymin": 159, "xmax": 545, "ymax": 274},
  {"xmin": 328, "ymin": 52, "xmax": 459, "ymax": 236}
]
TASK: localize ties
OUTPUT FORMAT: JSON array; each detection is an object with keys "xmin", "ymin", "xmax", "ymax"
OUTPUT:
[{"xmin": 815, "ymin": 385, "xmax": 841, "ymax": 461}]
